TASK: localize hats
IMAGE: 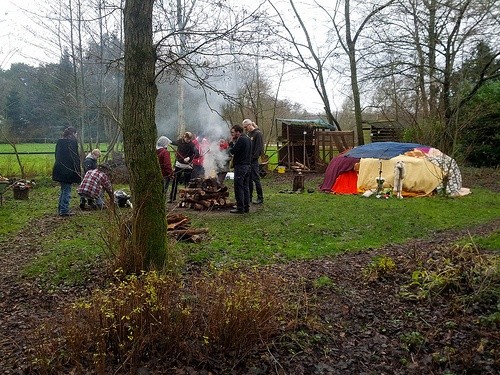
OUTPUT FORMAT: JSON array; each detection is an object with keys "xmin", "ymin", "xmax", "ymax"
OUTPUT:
[{"xmin": 156, "ymin": 136, "xmax": 172, "ymax": 149}]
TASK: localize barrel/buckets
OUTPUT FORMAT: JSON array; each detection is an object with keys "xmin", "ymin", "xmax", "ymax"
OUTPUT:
[{"xmin": 278, "ymin": 166, "xmax": 286, "ymax": 173}]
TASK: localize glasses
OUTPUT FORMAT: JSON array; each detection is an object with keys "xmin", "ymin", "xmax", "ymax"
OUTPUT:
[{"xmin": 244, "ymin": 123, "xmax": 251, "ymax": 128}]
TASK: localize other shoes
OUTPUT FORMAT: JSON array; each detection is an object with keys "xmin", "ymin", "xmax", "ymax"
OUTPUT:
[
  {"xmin": 94, "ymin": 205, "xmax": 106, "ymax": 210},
  {"xmin": 167, "ymin": 199, "xmax": 174, "ymax": 202},
  {"xmin": 230, "ymin": 209, "xmax": 244, "ymax": 213},
  {"xmin": 80, "ymin": 200, "xmax": 86, "ymax": 209},
  {"xmin": 60, "ymin": 211, "xmax": 75, "ymax": 216},
  {"xmin": 254, "ymin": 198, "xmax": 262, "ymax": 203}
]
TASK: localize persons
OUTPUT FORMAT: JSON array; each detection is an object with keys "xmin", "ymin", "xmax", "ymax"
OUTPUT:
[
  {"xmin": 52, "ymin": 127, "xmax": 80, "ymax": 215},
  {"xmin": 227, "ymin": 125, "xmax": 252, "ymax": 214},
  {"xmin": 154, "ymin": 119, "xmax": 266, "ymax": 204},
  {"xmin": 77, "ymin": 163, "xmax": 114, "ymax": 210},
  {"xmin": 82, "ymin": 149, "xmax": 108, "ymax": 209}
]
